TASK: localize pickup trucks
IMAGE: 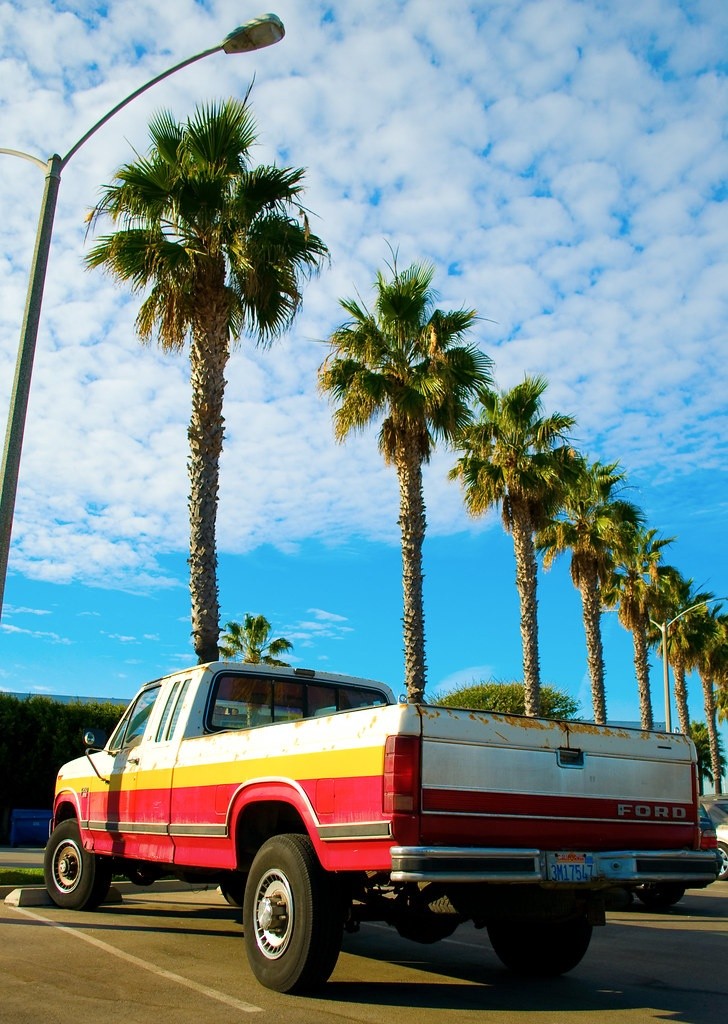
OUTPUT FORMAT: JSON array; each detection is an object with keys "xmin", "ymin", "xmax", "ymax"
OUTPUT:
[{"xmin": 42, "ymin": 660, "xmax": 704, "ymax": 994}]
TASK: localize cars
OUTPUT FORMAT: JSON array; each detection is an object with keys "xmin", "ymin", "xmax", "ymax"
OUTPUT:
[{"xmin": 631, "ymin": 793, "xmax": 728, "ymax": 908}]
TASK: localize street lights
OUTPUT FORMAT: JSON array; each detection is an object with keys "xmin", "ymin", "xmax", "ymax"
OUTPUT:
[
  {"xmin": 0, "ymin": 12, "xmax": 292, "ymax": 639},
  {"xmin": 599, "ymin": 595, "xmax": 728, "ymax": 734}
]
